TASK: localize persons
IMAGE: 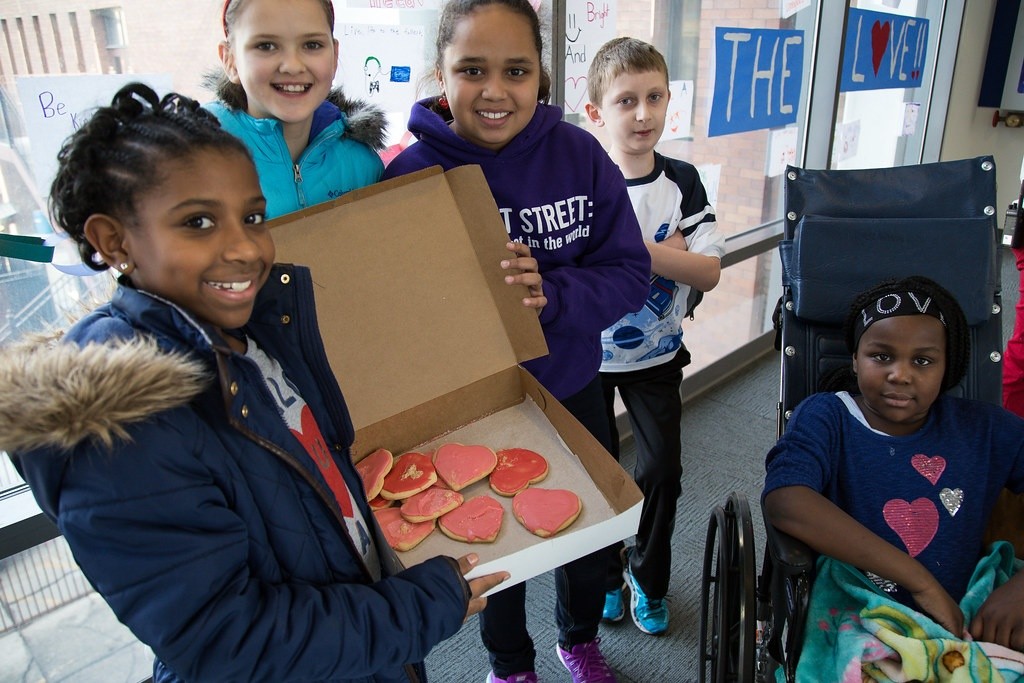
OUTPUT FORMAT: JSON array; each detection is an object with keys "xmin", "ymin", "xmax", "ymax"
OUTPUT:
[
  {"xmin": 1002, "ymin": 180, "xmax": 1024, "ymax": 423},
  {"xmin": 195, "ymin": 0, "xmax": 722, "ymax": 683},
  {"xmin": 2, "ymin": 81, "xmax": 510, "ymax": 683},
  {"xmin": 761, "ymin": 275, "xmax": 1024, "ymax": 683}
]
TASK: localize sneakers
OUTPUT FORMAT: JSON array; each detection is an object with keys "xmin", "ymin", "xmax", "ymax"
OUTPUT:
[
  {"xmin": 556, "ymin": 636, "xmax": 617, "ymax": 683},
  {"xmin": 599, "ymin": 580, "xmax": 627, "ymax": 626},
  {"xmin": 486, "ymin": 669, "xmax": 537, "ymax": 683},
  {"xmin": 620, "ymin": 544, "xmax": 677, "ymax": 635}
]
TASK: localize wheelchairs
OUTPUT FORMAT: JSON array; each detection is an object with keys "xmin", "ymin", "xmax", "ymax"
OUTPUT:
[{"xmin": 695, "ymin": 157, "xmax": 1005, "ymax": 683}]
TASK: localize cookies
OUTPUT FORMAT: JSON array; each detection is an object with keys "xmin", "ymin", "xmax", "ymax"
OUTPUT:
[{"xmin": 355, "ymin": 442, "xmax": 583, "ymax": 552}]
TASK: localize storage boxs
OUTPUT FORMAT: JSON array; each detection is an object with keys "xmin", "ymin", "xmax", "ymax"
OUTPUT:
[{"xmin": 265, "ymin": 163, "xmax": 646, "ymax": 598}]
